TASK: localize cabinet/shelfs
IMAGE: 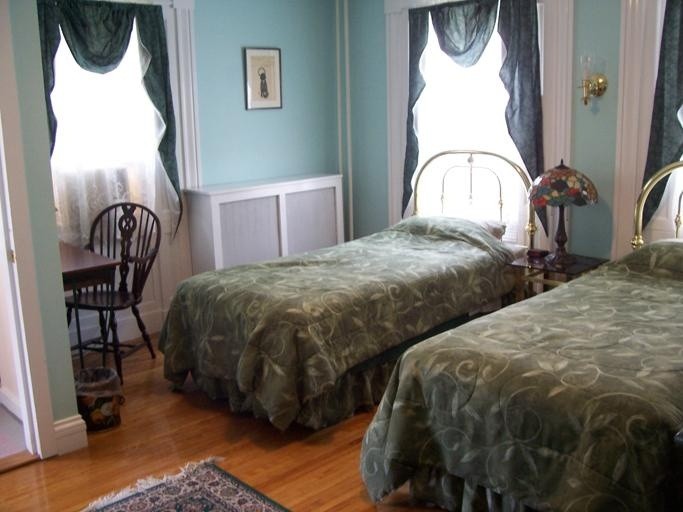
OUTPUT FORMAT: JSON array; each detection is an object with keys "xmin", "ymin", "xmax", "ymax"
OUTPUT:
[{"xmin": 181, "ymin": 175, "xmax": 348, "ymax": 277}]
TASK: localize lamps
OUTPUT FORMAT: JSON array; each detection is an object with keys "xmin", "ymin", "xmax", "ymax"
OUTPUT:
[
  {"xmin": 526, "ymin": 156, "xmax": 600, "ymax": 269},
  {"xmin": 579, "ymin": 50, "xmax": 608, "ymax": 106}
]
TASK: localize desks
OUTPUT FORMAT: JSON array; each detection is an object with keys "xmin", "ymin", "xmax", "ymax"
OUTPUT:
[{"xmin": 58, "ymin": 237, "xmax": 122, "ymax": 370}]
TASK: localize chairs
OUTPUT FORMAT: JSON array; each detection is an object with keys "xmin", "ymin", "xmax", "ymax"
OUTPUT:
[{"xmin": 59, "ymin": 201, "xmax": 161, "ymax": 387}]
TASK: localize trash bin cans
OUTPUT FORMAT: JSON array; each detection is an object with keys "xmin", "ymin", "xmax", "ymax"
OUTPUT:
[{"xmin": 74, "ymin": 367, "xmax": 121, "ymax": 432}]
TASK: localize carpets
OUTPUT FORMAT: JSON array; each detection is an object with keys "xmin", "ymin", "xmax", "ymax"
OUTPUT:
[{"xmin": 71, "ymin": 455, "xmax": 291, "ymax": 512}]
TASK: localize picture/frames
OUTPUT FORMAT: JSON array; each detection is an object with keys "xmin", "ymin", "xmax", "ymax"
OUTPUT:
[{"xmin": 241, "ymin": 45, "xmax": 285, "ymax": 112}]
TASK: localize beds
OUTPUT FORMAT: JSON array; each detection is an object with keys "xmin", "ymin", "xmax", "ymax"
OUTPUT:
[
  {"xmin": 387, "ymin": 159, "xmax": 682, "ymax": 510},
  {"xmin": 169, "ymin": 146, "xmax": 579, "ymax": 434}
]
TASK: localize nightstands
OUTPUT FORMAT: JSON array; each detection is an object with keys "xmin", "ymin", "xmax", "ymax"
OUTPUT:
[{"xmin": 508, "ymin": 252, "xmax": 608, "ymax": 304}]
